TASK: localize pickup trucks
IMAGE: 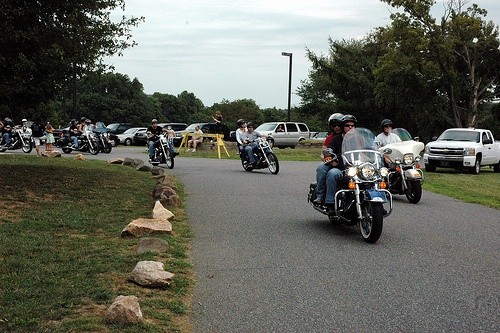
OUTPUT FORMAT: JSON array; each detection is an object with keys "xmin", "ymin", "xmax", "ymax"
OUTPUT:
[{"xmin": 423, "ymin": 128, "xmax": 500, "ymax": 175}]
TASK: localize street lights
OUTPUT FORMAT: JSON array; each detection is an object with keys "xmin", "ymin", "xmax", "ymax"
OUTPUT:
[{"xmin": 282, "ymin": 52, "xmax": 292, "ymax": 122}]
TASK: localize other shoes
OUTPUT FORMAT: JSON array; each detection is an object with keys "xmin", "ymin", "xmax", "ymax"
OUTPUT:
[
  {"xmin": 185, "ymin": 148, "xmax": 191, "ymax": 153},
  {"xmin": 172, "ymin": 151, "xmax": 179, "ymax": 157},
  {"xmin": 313, "ymin": 196, "xmax": 324, "ymax": 205},
  {"xmin": 326, "ymin": 205, "xmax": 335, "ymax": 216},
  {"xmin": 249, "ymin": 161, "xmax": 257, "ymax": 166},
  {"xmin": 191, "ymin": 149, "xmax": 197, "ymax": 153}
]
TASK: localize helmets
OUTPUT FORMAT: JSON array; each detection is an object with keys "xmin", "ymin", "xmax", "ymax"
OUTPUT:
[
  {"xmin": 5, "ymin": 117, "xmax": 12, "ymax": 122},
  {"xmin": 247, "ymin": 122, "xmax": 255, "ymax": 129},
  {"xmin": 328, "ymin": 113, "xmax": 344, "ymax": 126},
  {"xmin": 341, "ymin": 114, "xmax": 357, "ymax": 124},
  {"xmin": 237, "ymin": 119, "xmax": 246, "ymax": 126},
  {"xmin": 380, "ymin": 118, "xmax": 392, "ymax": 128},
  {"xmin": 69, "ymin": 117, "xmax": 91, "ymax": 124}
]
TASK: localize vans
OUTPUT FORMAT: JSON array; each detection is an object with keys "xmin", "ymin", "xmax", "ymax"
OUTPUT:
[{"xmin": 253, "ymin": 122, "xmax": 310, "ymax": 149}]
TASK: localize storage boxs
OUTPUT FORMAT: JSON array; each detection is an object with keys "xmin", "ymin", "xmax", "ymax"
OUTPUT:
[{"xmin": 57, "ymin": 137, "xmax": 66, "ymax": 143}]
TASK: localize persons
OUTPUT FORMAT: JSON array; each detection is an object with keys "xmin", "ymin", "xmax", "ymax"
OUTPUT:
[
  {"xmin": 70, "ymin": 117, "xmax": 113, "ymax": 147},
  {"xmin": 165, "ymin": 126, "xmax": 176, "ymax": 147},
  {"xmin": 372, "ymin": 119, "xmax": 402, "ymax": 169},
  {"xmin": 314, "ymin": 113, "xmax": 372, "ymax": 213},
  {"xmin": 276, "ymin": 125, "xmax": 284, "ymax": 132},
  {"xmin": 211, "ymin": 111, "xmax": 225, "ymax": 148},
  {"xmin": 187, "ymin": 125, "xmax": 203, "ymax": 153},
  {"xmin": 0, "ymin": 117, "xmax": 55, "ymax": 156},
  {"xmin": 236, "ymin": 119, "xmax": 269, "ymax": 165},
  {"xmin": 146, "ymin": 119, "xmax": 168, "ymax": 162}
]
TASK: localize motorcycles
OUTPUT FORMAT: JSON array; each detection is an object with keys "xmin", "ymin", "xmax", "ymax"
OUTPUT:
[
  {"xmin": 378, "ymin": 128, "xmax": 426, "ymax": 204},
  {"xmin": 145, "ymin": 131, "xmax": 176, "ymax": 169},
  {"xmin": 307, "ymin": 128, "xmax": 394, "ymax": 244},
  {"xmin": 0, "ymin": 122, "xmax": 113, "ymax": 155},
  {"xmin": 237, "ymin": 136, "xmax": 280, "ymax": 175}
]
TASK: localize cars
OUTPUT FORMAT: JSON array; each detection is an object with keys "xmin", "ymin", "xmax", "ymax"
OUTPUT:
[
  {"xmin": 106, "ymin": 123, "xmax": 132, "ymax": 134},
  {"xmin": 116, "ymin": 128, "xmax": 148, "ymax": 146}
]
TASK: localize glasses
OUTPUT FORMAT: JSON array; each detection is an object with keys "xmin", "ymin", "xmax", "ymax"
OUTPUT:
[
  {"xmin": 343, "ymin": 124, "xmax": 355, "ymax": 127},
  {"xmin": 249, "ymin": 126, "xmax": 253, "ymax": 128},
  {"xmin": 385, "ymin": 125, "xmax": 391, "ymax": 127}
]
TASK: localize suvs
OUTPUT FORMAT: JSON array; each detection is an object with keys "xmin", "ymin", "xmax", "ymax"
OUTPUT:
[
  {"xmin": 174, "ymin": 123, "xmax": 230, "ymax": 144},
  {"xmin": 134, "ymin": 123, "xmax": 189, "ymax": 143}
]
